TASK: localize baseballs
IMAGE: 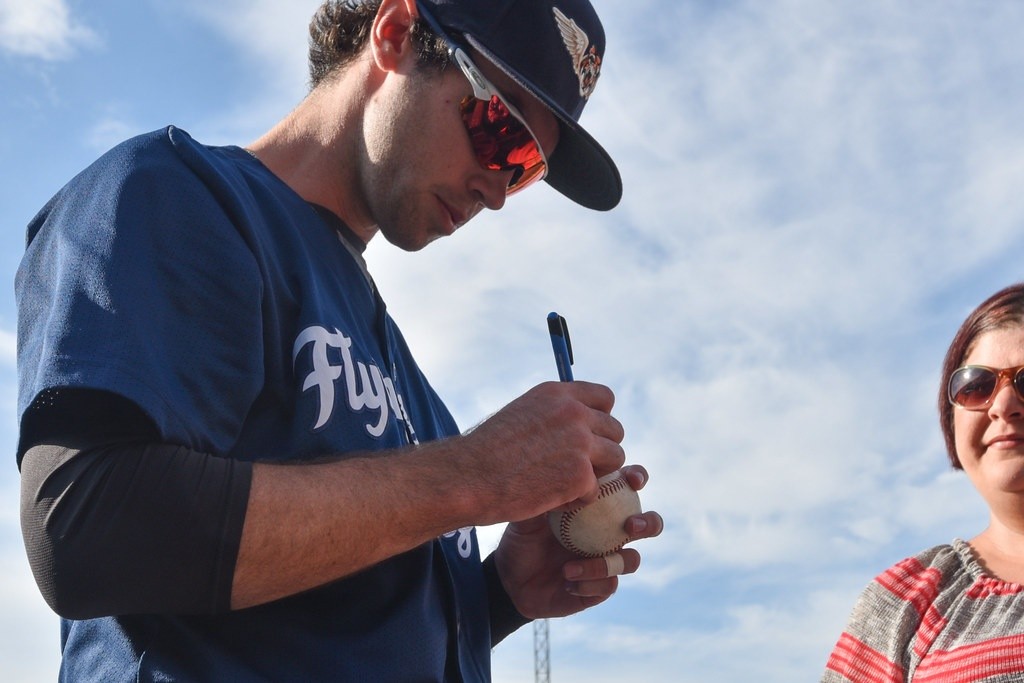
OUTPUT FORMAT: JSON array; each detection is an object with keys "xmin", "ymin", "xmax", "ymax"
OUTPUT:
[{"xmin": 549, "ymin": 469, "xmax": 643, "ymax": 557}]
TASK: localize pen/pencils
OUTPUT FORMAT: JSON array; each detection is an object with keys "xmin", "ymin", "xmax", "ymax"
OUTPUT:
[{"xmin": 547, "ymin": 311, "xmax": 574, "ymax": 381}]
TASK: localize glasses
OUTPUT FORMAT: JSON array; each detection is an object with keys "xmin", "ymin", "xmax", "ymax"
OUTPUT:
[
  {"xmin": 947, "ymin": 365, "xmax": 1024, "ymax": 409},
  {"xmin": 417, "ymin": 0, "xmax": 548, "ymax": 198}
]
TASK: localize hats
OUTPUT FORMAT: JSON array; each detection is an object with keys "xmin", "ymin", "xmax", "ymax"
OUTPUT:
[{"xmin": 421, "ymin": 0, "xmax": 623, "ymax": 212}]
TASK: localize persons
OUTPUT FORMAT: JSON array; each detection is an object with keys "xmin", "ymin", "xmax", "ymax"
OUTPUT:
[
  {"xmin": 15, "ymin": 0, "xmax": 664, "ymax": 683},
  {"xmin": 817, "ymin": 282, "xmax": 1024, "ymax": 683}
]
